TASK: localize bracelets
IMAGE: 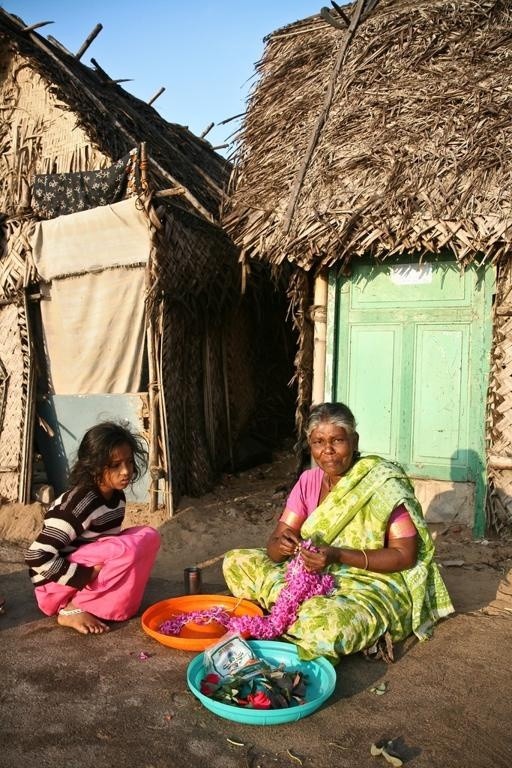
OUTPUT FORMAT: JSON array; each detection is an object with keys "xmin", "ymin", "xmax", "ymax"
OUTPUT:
[{"xmin": 360, "ymin": 550, "xmax": 368, "ymax": 570}]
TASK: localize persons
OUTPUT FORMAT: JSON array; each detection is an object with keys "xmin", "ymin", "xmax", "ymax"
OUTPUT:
[
  {"xmin": 23, "ymin": 423, "xmax": 161, "ymax": 635},
  {"xmin": 222, "ymin": 404, "xmax": 456, "ymax": 665}
]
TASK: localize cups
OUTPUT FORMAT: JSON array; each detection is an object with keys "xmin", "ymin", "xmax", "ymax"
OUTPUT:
[{"xmin": 184, "ymin": 567, "xmax": 202, "ymax": 595}]
